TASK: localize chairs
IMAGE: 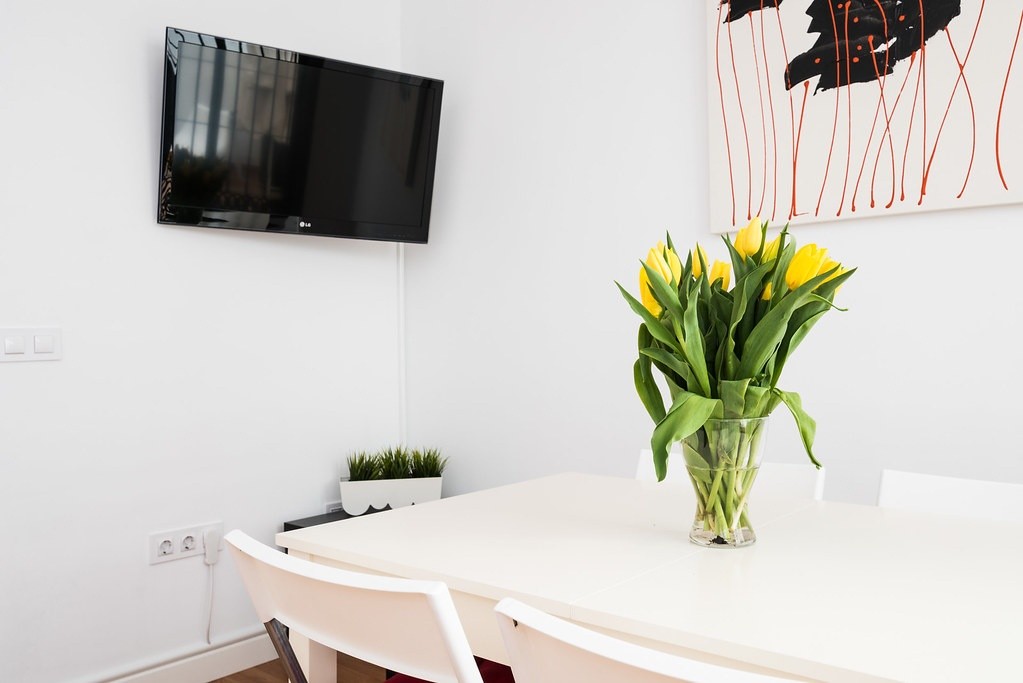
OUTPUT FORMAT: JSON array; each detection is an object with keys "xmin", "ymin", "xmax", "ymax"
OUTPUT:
[
  {"xmin": 221, "ymin": 529, "xmax": 485, "ymax": 683},
  {"xmin": 493, "ymin": 599, "xmax": 811, "ymax": 683}
]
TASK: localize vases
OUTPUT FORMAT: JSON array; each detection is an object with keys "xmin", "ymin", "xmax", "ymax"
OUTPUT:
[{"xmin": 675, "ymin": 415, "xmax": 769, "ymax": 546}]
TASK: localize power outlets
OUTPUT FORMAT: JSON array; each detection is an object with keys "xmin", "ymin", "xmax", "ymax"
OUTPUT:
[{"xmin": 147, "ymin": 522, "xmax": 225, "ymax": 566}]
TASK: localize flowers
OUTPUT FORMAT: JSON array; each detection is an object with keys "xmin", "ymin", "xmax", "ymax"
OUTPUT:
[{"xmin": 613, "ymin": 214, "xmax": 858, "ymax": 542}]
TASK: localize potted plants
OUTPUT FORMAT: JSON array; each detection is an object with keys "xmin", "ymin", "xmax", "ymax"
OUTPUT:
[{"xmin": 338, "ymin": 445, "xmax": 452, "ymax": 515}]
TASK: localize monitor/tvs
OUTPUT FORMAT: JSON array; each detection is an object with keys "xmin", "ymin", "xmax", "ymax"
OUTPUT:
[{"xmin": 156, "ymin": 27, "xmax": 445, "ymax": 245}]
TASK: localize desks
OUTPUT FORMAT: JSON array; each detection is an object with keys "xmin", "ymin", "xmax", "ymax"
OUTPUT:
[
  {"xmin": 285, "ymin": 504, "xmax": 417, "ymax": 680},
  {"xmin": 274, "ymin": 472, "xmax": 1023, "ymax": 683}
]
